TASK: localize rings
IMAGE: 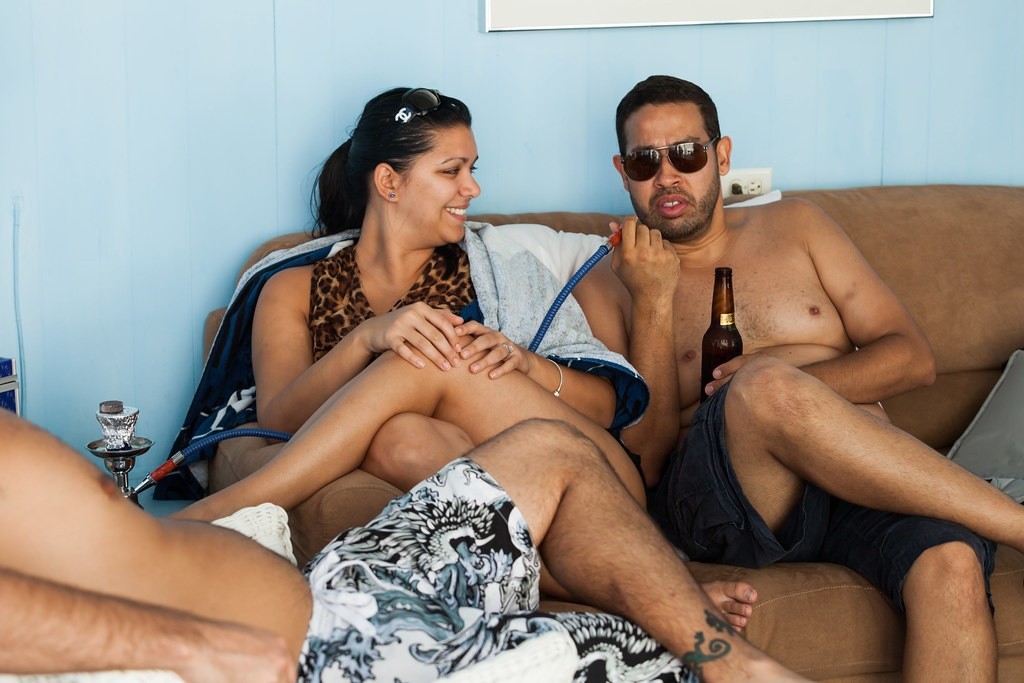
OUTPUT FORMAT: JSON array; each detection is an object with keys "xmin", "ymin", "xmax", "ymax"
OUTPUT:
[{"xmin": 502, "ymin": 344, "xmax": 514, "ymax": 360}]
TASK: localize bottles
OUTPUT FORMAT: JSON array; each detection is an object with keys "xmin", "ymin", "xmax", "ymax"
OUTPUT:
[{"xmin": 700, "ymin": 267, "xmax": 743, "ymax": 403}]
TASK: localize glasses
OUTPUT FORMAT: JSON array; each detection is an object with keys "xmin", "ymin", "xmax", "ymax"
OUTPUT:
[
  {"xmin": 621, "ymin": 135, "xmax": 719, "ymax": 181},
  {"xmin": 373, "ymin": 87, "xmax": 442, "ymax": 156}
]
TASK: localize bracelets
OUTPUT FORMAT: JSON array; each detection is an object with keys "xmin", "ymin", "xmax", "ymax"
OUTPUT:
[{"xmin": 547, "ymin": 358, "xmax": 563, "ymax": 397}]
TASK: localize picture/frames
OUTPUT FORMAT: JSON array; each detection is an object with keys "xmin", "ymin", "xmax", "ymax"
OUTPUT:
[{"xmin": 485, "ymin": 0, "xmax": 933, "ymax": 32}]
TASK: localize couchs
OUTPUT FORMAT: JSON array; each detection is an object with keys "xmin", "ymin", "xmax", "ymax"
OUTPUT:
[{"xmin": 201, "ymin": 186, "xmax": 1024, "ymax": 683}]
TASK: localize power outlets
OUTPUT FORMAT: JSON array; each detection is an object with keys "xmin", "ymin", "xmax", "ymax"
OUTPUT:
[{"xmin": 727, "ymin": 167, "xmax": 772, "ymax": 195}]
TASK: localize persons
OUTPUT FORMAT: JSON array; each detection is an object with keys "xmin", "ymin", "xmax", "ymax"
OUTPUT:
[
  {"xmin": 573, "ymin": 76, "xmax": 1024, "ymax": 683},
  {"xmin": 0, "ymin": 413, "xmax": 815, "ymax": 683},
  {"xmin": 167, "ymin": 84, "xmax": 760, "ymax": 627}
]
